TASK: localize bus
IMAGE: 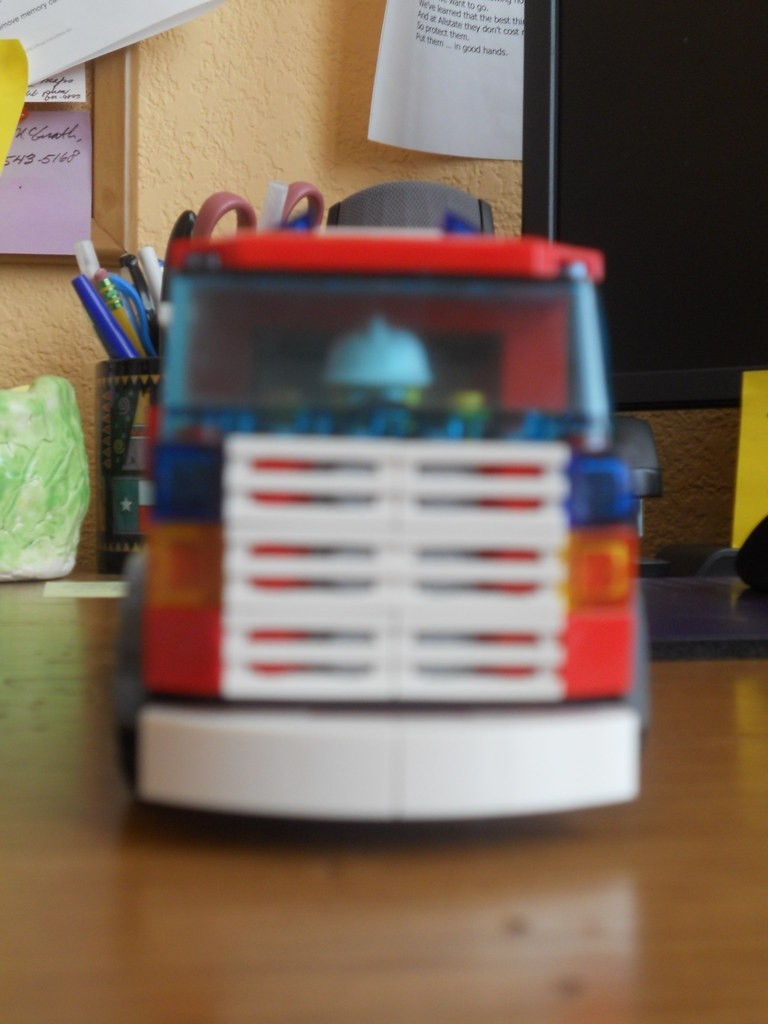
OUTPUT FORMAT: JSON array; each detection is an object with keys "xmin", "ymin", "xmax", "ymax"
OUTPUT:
[{"xmin": 113, "ymin": 231, "xmax": 662, "ymax": 822}]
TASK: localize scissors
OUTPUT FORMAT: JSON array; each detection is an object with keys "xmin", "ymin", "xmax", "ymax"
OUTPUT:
[{"xmin": 191, "ymin": 181, "xmax": 324, "ymax": 247}]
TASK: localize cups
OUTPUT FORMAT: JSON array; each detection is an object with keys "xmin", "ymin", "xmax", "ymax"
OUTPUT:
[{"xmin": 96, "ymin": 358, "xmax": 160, "ymax": 575}]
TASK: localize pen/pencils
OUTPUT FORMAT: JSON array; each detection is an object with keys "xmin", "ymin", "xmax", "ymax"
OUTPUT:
[{"xmin": 70, "ymin": 210, "xmax": 197, "ymax": 359}]
